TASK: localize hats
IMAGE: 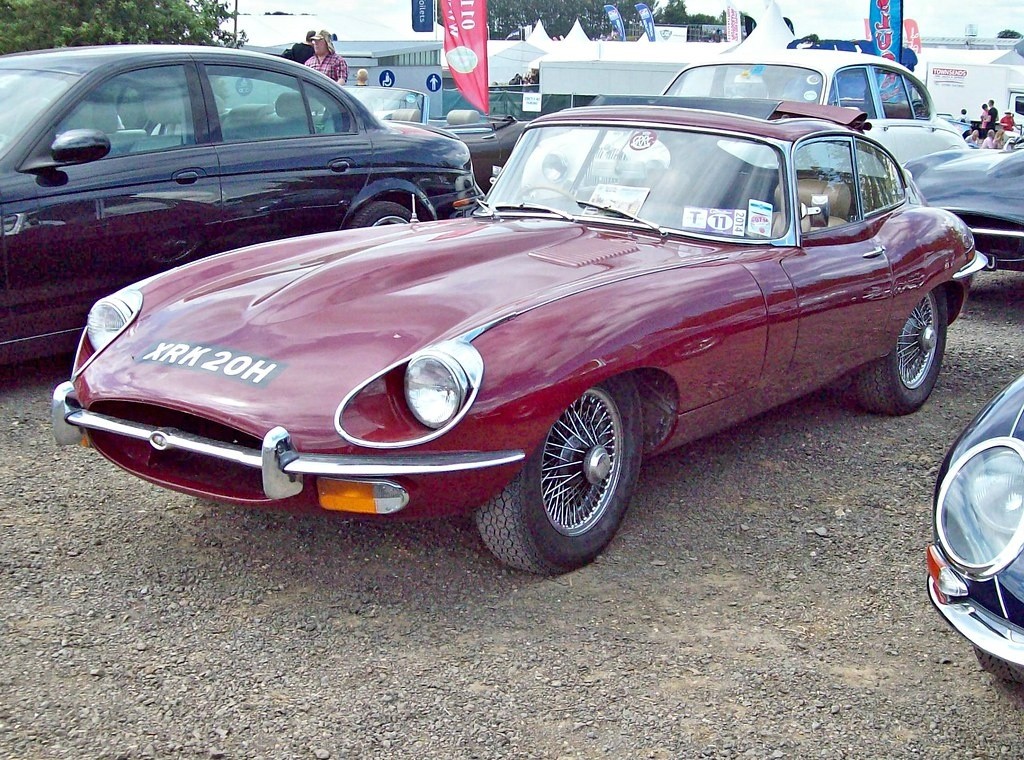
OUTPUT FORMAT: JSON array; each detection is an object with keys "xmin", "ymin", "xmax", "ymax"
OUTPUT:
[{"xmin": 310, "ymin": 30, "xmax": 331, "ymax": 40}]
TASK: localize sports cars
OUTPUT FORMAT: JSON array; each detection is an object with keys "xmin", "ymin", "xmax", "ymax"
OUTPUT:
[
  {"xmin": 894, "ymin": 144, "xmax": 1024, "ymax": 276},
  {"xmin": 328, "ymin": 83, "xmax": 541, "ymax": 198},
  {"xmin": 51, "ymin": 95, "xmax": 986, "ymax": 578}
]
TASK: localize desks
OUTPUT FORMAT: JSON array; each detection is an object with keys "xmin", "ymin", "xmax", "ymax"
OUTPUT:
[{"xmin": 970, "ymin": 121, "xmax": 1000, "ymax": 127}]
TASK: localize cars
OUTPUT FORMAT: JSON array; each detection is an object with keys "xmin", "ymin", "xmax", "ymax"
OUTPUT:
[
  {"xmin": 923, "ymin": 372, "xmax": 1024, "ymax": 691},
  {"xmin": 0, "ymin": 43, "xmax": 488, "ymax": 371},
  {"xmin": 659, "ymin": 49, "xmax": 970, "ymax": 172}
]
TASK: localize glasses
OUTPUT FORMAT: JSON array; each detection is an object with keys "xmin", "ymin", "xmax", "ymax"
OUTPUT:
[{"xmin": 312, "ymin": 40, "xmax": 324, "ymax": 45}]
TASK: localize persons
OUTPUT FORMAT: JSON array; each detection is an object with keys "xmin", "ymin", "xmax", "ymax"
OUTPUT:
[
  {"xmin": 356, "ymin": 69, "xmax": 368, "ymax": 86},
  {"xmin": 956, "ymin": 100, "xmax": 1014, "ymax": 149},
  {"xmin": 292, "ymin": 30, "xmax": 316, "ymax": 64},
  {"xmin": 305, "ymin": 30, "xmax": 348, "ymax": 115},
  {"xmin": 492, "ymin": 73, "xmax": 538, "ymax": 91}
]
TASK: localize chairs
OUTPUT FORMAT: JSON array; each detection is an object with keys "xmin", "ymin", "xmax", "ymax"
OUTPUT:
[
  {"xmin": 745, "ymin": 179, "xmax": 851, "ymax": 237},
  {"xmin": 392, "ymin": 109, "xmax": 422, "ymax": 123},
  {"xmin": 60, "ymin": 95, "xmax": 120, "ymax": 148},
  {"xmin": 213, "ymin": 91, "xmax": 317, "ymax": 140},
  {"xmin": 129, "ymin": 89, "xmax": 183, "ymax": 151},
  {"xmin": 446, "ymin": 109, "xmax": 480, "ymax": 125}
]
